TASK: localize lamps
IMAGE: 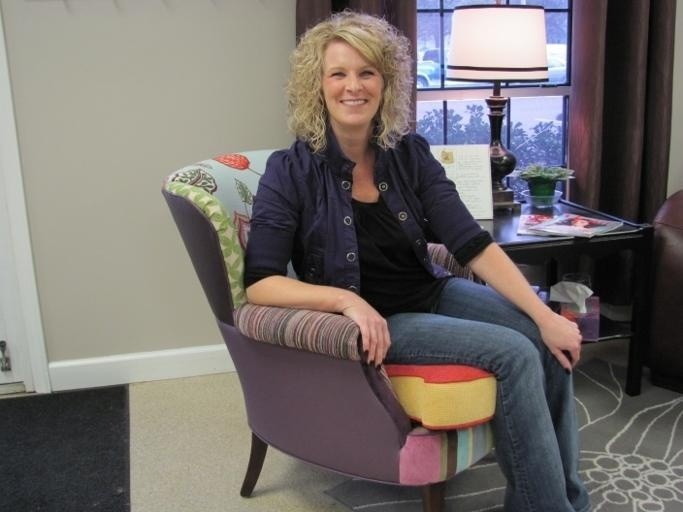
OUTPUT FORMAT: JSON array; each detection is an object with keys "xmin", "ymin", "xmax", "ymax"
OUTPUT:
[{"xmin": 445, "ymin": 5, "xmax": 549, "ymax": 188}]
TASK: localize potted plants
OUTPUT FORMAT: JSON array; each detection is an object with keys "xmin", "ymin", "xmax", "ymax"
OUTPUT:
[{"xmin": 517, "ymin": 161, "xmax": 574, "ymax": 195}]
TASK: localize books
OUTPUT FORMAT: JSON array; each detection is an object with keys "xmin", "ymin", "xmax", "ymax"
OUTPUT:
[
  {"xmin": 527, "ymin": 211, "xmax": 626, "ymax": 238},
  {"xmin": 516, "ymin": 212, "xmax": 575, "ymax": 241}
]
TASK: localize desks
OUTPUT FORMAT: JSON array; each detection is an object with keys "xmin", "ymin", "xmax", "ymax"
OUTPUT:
[{"xmin": 475, "ymin": 200, "xmax": 654, "ymax": 396}]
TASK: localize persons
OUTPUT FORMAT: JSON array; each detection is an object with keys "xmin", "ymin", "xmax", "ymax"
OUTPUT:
[
  {"xmin": 536, "ymin": 214, "xmax": 552, "ymax": 225},
  {"xmin": 558, "ymin": 216, "xmax": 595, "ymax": 229},
  {"xmin": 240, "ymin": 10, "xmax": 595, "ymax": 512}
]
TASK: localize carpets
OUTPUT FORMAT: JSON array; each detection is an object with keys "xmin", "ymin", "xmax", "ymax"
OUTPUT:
[
  {"xmin": 319, "ymin": 328, "xmax": 683, "ymax": 512},
  {"xmin": 0, "ymin": 383, "xmax": 132, "ymax": 512}
]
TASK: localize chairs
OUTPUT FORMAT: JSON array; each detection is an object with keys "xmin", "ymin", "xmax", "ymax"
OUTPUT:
[
  {"xmin": 162, "ymin": 142, "xmax": 498, "ymax": 512},
  {"xmin": 639, "ymin": 188, "xmax": 683, "ymax": 393}
]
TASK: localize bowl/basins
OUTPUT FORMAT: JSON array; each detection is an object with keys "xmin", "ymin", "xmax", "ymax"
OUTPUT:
[{"xmin": 520, "ymin": 189, "xmax": 563, "ymax": 207}]
